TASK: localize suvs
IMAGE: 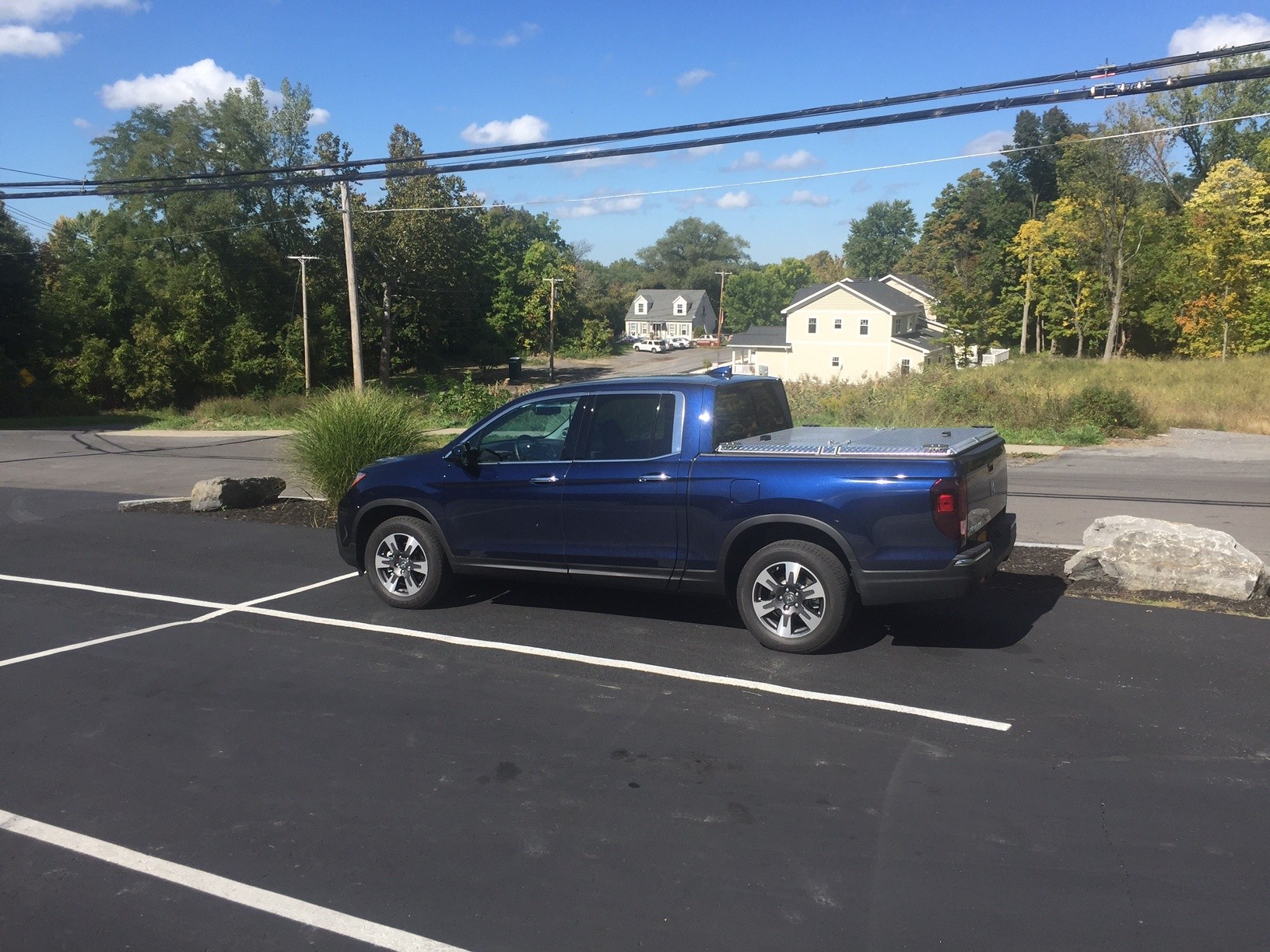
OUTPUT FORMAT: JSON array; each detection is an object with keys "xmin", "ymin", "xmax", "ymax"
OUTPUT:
[{"xmin": 632, "ymin": 340, "xmax": 666, "ymax": 353}]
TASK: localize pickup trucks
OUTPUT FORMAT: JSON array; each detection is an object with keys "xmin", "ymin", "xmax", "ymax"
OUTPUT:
[
  {"xmin": 665, "ymin": 338, "xmax": 684, "ymax": 348},
  {"xmin": 343, "ymin": 363, "xmax": 1017, "ymax": 656}
]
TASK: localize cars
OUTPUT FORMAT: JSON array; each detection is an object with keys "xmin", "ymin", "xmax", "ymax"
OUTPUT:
[
  {"xmin": 665, "ymin": 341, "xmax": 674, "ymax": 351},
  {"xmin": 692, "ymin": 335, "xmax": 719, "ymax": 347},
  {"xmin": 677, "ymin": 337, "xmax": 692, "ymax": 347}
]
